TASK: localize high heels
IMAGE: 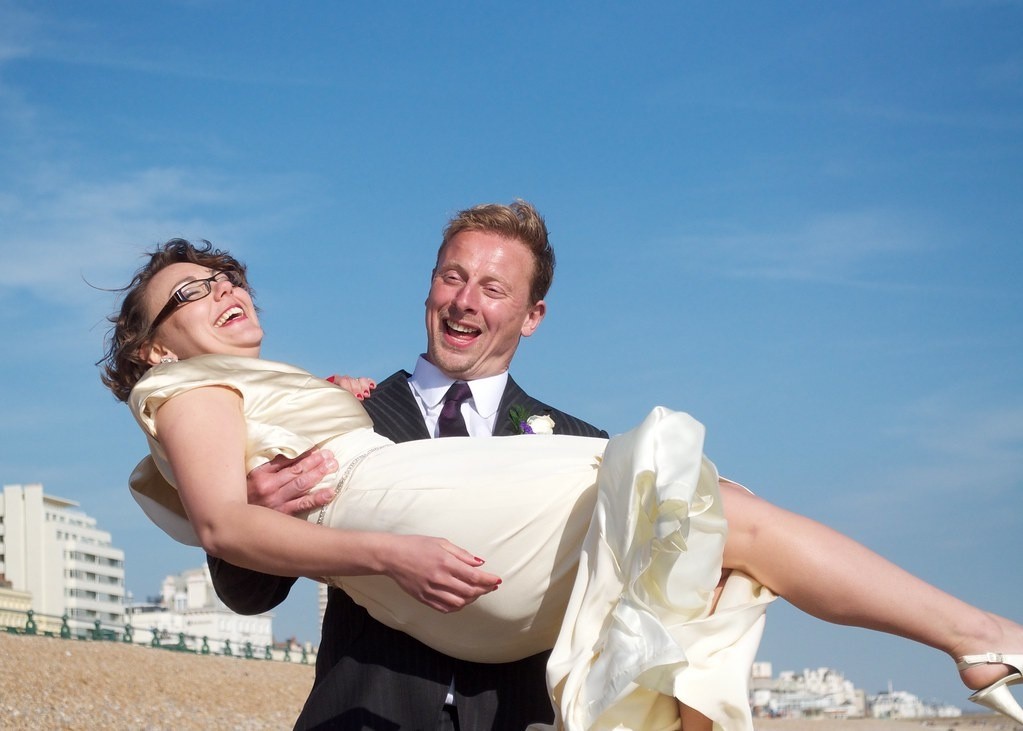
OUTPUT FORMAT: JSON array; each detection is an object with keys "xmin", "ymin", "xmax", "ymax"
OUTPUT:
[{"xmin": 956, "ymin": 652, "xmax": 1023, "ymax": 725}]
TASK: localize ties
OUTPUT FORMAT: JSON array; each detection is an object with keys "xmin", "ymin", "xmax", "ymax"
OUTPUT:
[{"xmin": 438, "ymin": 382, "xmax": 472, "ymax": 437}]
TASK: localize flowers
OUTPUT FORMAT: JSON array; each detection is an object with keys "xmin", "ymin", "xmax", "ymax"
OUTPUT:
[{"xmin": 506, "ymin": 404, "xmax": 554, "ymax": 436}]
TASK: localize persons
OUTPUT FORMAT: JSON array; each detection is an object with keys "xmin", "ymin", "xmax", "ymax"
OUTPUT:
[
  {"xmin": 206, "ymin": 197, "xmax": 611, "ymax": 730},
  {"xmin": 93, "ymin": 237, "xmax": 1023, "ymax": 731}
]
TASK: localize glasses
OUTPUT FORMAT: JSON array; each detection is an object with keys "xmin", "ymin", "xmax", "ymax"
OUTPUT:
[{"xmin": 147, "ymin": 268, "xmax": 243, "ymax": 336}]
